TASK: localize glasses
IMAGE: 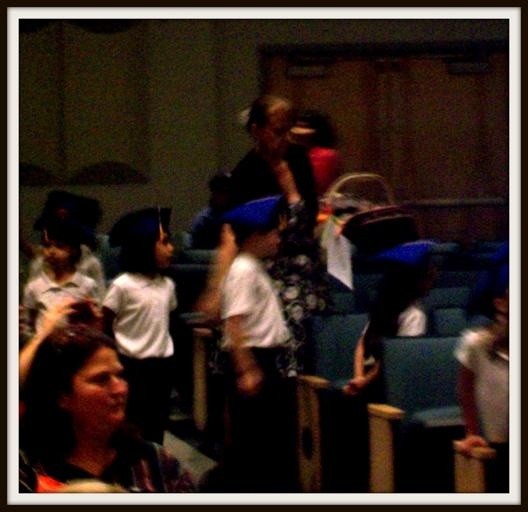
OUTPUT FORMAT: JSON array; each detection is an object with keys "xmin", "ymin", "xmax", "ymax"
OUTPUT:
[{"xmin": 332, "ymin": 205, "xmax": 358, "ymax": 215}]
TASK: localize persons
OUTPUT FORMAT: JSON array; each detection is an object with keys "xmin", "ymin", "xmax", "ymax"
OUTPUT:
[
  {"xmin": 191, "ymin": 213, "xmax": 307, "ymax": 493},
  {"xmin": 209, "ymin": 88, "xmax": 481, "ymax": 394},
  {"xmin": 446, "ymin": 277, "xmax": 510, "ymax": 494},
  {"xmin": 16, "ymin": 322, "xmax": 193, "ymax": 495},
  {"xmin": 97, "ymin": 223, "xmax": 176, "ymax": 453},
  {"xmin": 21, "ymin": 227, "xmax": 101, "ymax": 331}
]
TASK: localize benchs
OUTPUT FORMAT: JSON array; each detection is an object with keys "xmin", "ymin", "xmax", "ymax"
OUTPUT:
[{"xmin": 95, "ymin": 228, "xmax": 509, "ymax": 493}]
